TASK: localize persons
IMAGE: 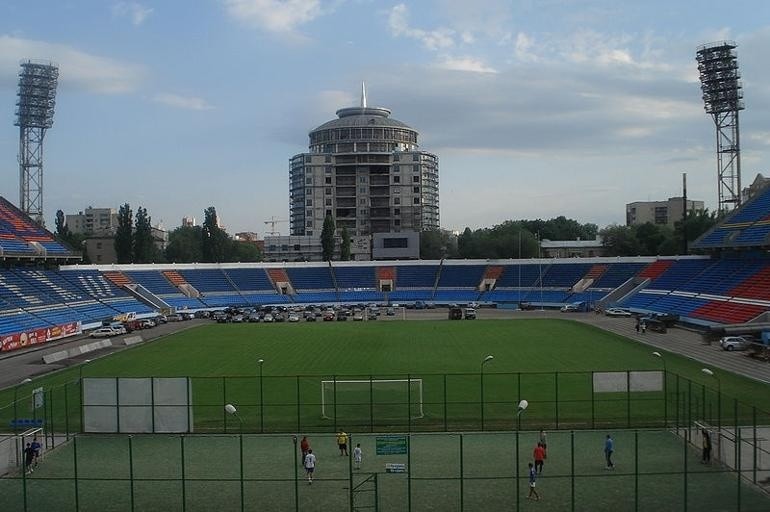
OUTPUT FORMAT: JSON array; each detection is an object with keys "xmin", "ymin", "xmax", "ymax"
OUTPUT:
[
  {"xmin": 700, "ymin": 428, "xmax": 712, "ymax": 465},
  {"xmin": 525, "ymin": 463, "xmax": 541, "ymax": 502},
  {"xmin": 604, "ymin": 435, "xmax": 616, "ymax": 470},
  {"xmin": 304, "ymin": 450, "xmax": 316, "ymax": 484},
  {"xmin": 539, "ymin": 430, "xmax": 548, "ymax": 458},
  {"xmin": 24, "ymin": 442, "xmax": 34, "ymax": 475},
  {"xmin": 636, "ymin": 321, "xmax": 640, "ymax": 333},
  {"xmin": 336, "ymin": 428, "xmax": 349, "ymax": 456},
  {"xmin": 353, "ymin": 444, "xmax": 362, "ymax": 470},
  {"xmin": 533, "ymin": 442, "xmax": 545, "ymax": 474},
  {"xmin": 301, "ymin": 437, "xmax": 309, "ymax": 465},
  {"xmin": 31, "ymin": 439, "xmax": 41, "ymax": 468},
  {"xmin": 641, "ymin": 322, "xmax": 647, "ymax": 334}
]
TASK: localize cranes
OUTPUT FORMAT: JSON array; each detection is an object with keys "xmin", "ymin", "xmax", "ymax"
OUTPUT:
[{"xmin": 263, "ymin": 214, "xmax": 290, "ymax": 237}]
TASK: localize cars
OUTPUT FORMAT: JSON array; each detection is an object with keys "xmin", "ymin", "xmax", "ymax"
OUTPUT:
[
  {"xmin": 559, "ymin": 300, "xmax": 752, "ymax": 351},
  {"xmin": 88, "ymin": 311, "xmax": 194, "ymax": 339},
  {"xmin": 197, "ymin": 301, "xmax": 485, "ymax": 324}
]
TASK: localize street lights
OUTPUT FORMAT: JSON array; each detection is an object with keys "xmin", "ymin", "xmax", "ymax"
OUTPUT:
[
  {"xmin": 224, "ymin": 400, "xmax": 246, "ymax": 512},
  {"xmin": 515, "ymin": 398, "xmax": 529, "ymax": 510},
  {"xmin": 12, "ymin": 376, "xmax": 34, "ymax": 464},
  {"xmin": 79, "ymin": 357, "xmax": 92, "ymax": 434},
  {"xmin": 257, "ymin": 358, "xmax": 267, "ymax": 433},
  {"xmin": 479, "ymin": 353, "xmax": 495, "ymax": 431},
  {"xmin": 654, "ymin": 351, "xmax": 668, "ymax": 428},
  {"xmin": 702, "ymin": 367, "xmax": 723, "ymax": 467}
]
